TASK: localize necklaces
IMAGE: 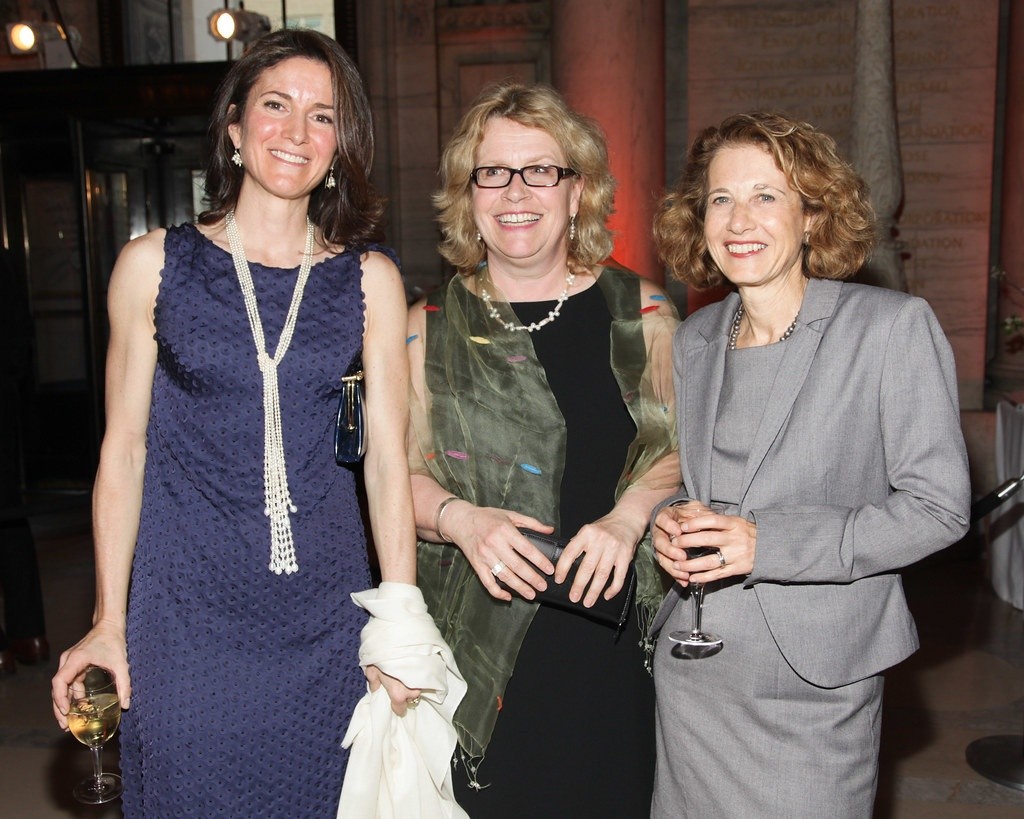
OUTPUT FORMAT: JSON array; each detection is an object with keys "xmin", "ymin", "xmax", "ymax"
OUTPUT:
[
  {"xmin": 479, "ymin": 258, "xmax": 574, "ymax": 331},
  {"xmin": 221, "ymin": 206, "xmax": 316, "ymax": 578},
  {"xmin": 729, "ymin": 302, "xmax": 800, "ymax": 351}
]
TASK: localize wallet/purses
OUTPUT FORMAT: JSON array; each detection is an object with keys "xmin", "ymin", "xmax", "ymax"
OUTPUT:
[{"xmin": 499, "ymin": 525, "xmax": 637, "ymax": 629}]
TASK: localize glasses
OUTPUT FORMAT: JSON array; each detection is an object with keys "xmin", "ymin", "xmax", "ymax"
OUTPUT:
[{"xmin": 468, "ymin": 165, "xmax": 583, "ymax": 188}]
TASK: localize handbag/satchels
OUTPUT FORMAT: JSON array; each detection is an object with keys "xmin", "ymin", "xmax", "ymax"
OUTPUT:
[{"xmin": 334, "ymin": 367, "xmax": 369, "ymax": 463}]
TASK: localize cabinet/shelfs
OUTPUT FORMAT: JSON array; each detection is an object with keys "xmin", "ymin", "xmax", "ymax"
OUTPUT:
[{"xmin": 0, "ymin": 59, "xmax": 260, "ymax": 677}]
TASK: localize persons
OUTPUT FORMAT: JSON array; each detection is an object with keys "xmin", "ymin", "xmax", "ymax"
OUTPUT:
[
  {"xmin": 402, "ymin": 77, "xmax": 682, "ymax": 819},
  {"xmin": 51, "ymin": 28, "xmax": 424, "ymax": 819},
  {"xmin": 649, "ymin": 111, "xmax": 972, "ymax": 819}
]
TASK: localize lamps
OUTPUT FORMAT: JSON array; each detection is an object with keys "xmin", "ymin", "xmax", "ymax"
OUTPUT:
[
  {"xmin": 10, "ymin": 21, "xmax": 81, "ymax": 68},
  {"xmin": 207, "ymin": 9, "xmax": 271, "ymax": 44}
]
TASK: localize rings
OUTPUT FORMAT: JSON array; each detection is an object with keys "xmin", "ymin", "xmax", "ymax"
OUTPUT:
[
  {"xmin": 717, "ymin": 551, "xmax": 726, "ymax": 567},
  {"xmin": 490, "ymin": 561, "xmax": 505, "ymax": 576},
  {"xmin": 406, "ymin": 696, "xmax": 421, "ymax": 706}
]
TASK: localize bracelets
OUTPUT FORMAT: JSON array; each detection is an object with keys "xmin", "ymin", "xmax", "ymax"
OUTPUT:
[{"xmin": 434, "ymin": 497, "xmax": 461, "ymax": 542}]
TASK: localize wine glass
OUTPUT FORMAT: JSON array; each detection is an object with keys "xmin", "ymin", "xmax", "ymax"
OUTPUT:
[
  {"xmin": 668, "ymin": 502, "xmax": 725, "ymax": 646},
  {"xmin": 67, "ymin": 667, "xmax": 125, "ymax": 804}
]
